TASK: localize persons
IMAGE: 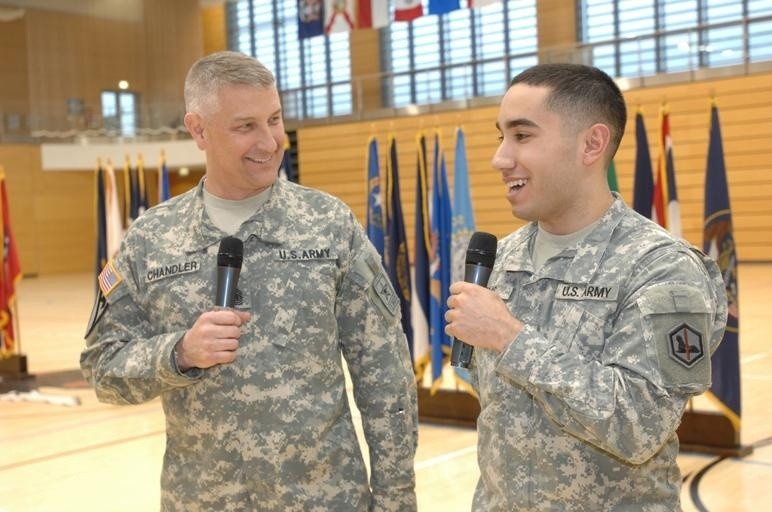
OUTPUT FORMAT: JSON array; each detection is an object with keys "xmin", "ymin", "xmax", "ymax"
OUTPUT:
[
  {"xmin": 441, "ymin": 62, "xmax": 731, "ymax": 512},
  {"xmin": 76, "ymin": 53, "xmax": 421, "ymax": 512}
]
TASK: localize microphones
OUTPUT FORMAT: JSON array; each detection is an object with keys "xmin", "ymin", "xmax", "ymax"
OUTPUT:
[
  {"xmin": 450, "ymin": 232, "xmax": 497, "ymax": 368},
  {"xmin": 215, "ymin": 237, "xmax": 243, "ymax": 308}
]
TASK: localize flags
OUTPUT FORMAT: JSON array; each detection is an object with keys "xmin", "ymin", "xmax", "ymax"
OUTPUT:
[
  {"xmin": 363, "ymin": 122, "xmax": 476, "ymax": 372},
  {"xmin": 93, "ymin": 134, "xmax": 294, "ymax": 279},
  {"xmin": 0, "ymin": 166, "xmax": 26, "ymax": 357},
  {"xmin": 294, "ymin": 1, "xmax": 505, "ymax": 42},
  {"xmin": 604, "ymin": 94, "xmax": 743, "ymax": 419}
]
